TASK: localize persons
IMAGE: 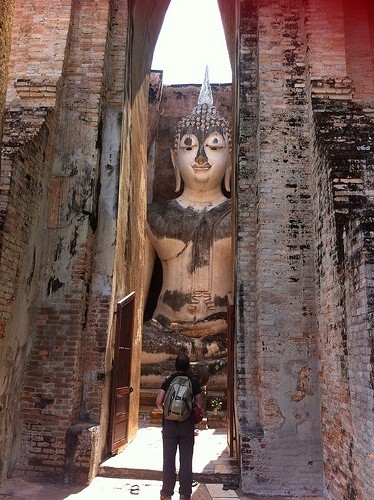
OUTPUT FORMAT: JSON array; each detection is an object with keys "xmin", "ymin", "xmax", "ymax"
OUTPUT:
[
  {"xmin": 156, "ymin": 353, "xmax": 203, "ymax": 500},
  {"xmin": 143, "ymin": 104, "xmax": 232, "ymax": 353}
]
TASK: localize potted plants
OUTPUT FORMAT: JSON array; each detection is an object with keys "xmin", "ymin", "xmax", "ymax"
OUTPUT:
[{"xmin": 210, "ymin": 397, "xmax": 223, "ymax": 415}]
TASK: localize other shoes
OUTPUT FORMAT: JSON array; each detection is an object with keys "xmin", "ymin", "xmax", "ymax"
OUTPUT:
[
  {"xmin": 160, "ymin": 494, "xmax": 171, "ymax": 500},
  {"xmin": 179, "ymin": 494, "xmax": 190, "ymax": 500}
]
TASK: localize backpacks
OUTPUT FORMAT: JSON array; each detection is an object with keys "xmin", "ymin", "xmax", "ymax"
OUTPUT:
[{"xmin": 164, "ymin": 370, "xmax": 196, "ymax": 423}]
